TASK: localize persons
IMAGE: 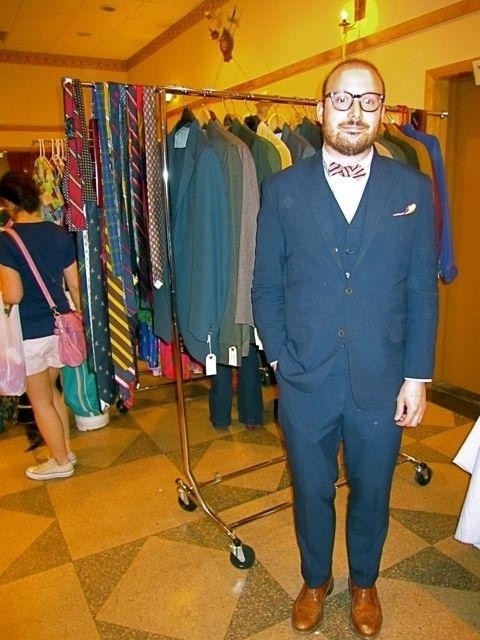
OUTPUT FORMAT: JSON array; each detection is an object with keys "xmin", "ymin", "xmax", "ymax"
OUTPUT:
[
  {"xmin": 251, "ymin": 59, "xmax": 440, "ymax": 640},
  {"xmin": 210, "ymin": 347, "xmax": 264, "ymax": 430},
  {"xmin": 0, "ymin": 171, "xmax": 81, "ymax": 481}
]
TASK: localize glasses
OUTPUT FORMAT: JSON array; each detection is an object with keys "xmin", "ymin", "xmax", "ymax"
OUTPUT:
[{"xmin": 324, "ymin": 91, "xmax": 385, "ymax": 112}]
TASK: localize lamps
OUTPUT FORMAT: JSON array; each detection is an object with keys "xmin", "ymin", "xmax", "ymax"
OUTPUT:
[{"xmin": 335, "ymin": 0, "xmax": 367, "ymax": 63}]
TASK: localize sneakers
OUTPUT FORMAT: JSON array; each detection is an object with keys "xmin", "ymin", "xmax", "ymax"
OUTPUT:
[
  {"xmin": 68, "ymin": 452, "xmax": 77, "ymax": 466},
  {"xmin": 25, "ymin": 456, "xmax": 74, "ymax": 480}
]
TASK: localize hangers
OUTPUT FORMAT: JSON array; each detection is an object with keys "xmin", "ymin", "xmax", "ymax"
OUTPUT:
[
  {"xmin": 179, "ymin": 87, "xmax": 415, "ymax": 133},
  {"xmin": 35, "ymin": 137, "xmax": 67, "ymax": 180}
]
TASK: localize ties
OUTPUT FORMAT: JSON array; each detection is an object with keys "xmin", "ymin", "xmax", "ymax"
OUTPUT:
[{"xmin": 61, "ymin": 73, "xmax": 171, "ymax": 412}]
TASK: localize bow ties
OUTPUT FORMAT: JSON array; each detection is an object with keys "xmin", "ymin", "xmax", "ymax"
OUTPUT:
[{"xmin": 328, "ymin": 161, "xmax": 366, "ymax": 178}]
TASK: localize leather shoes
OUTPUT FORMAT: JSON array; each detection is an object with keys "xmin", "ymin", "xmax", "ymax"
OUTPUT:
[
  {"xmin": 347, "ymin": 575, "xmax": 383, "ymax": 640},
  {"xmin": 291, "ymin": 576, "xmax": 334, "ymax": 634}
]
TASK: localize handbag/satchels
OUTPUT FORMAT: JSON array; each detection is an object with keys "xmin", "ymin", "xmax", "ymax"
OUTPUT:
[{"xmin": 54, "ymin": 312, "xmax": 87, "ymax": 367}]
